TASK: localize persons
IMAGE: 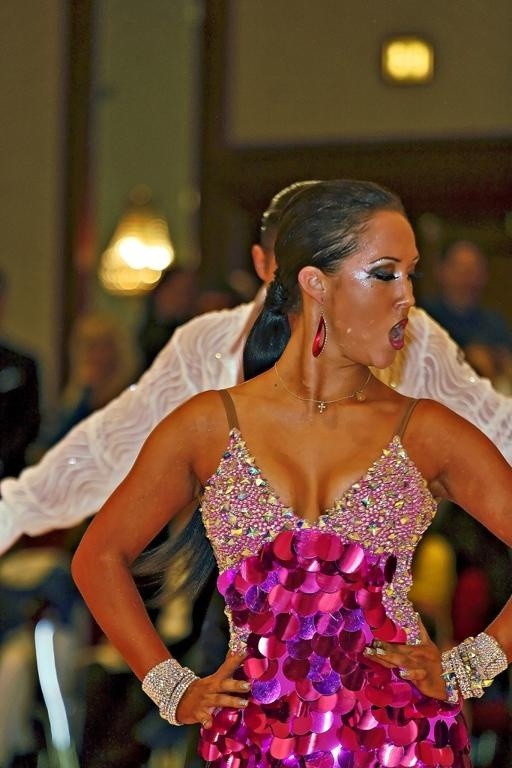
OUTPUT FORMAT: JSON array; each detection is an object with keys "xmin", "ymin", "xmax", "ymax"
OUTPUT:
[{"xmin": 1, "ymin": 232, "xmax": 512, "ymax": 768}]
